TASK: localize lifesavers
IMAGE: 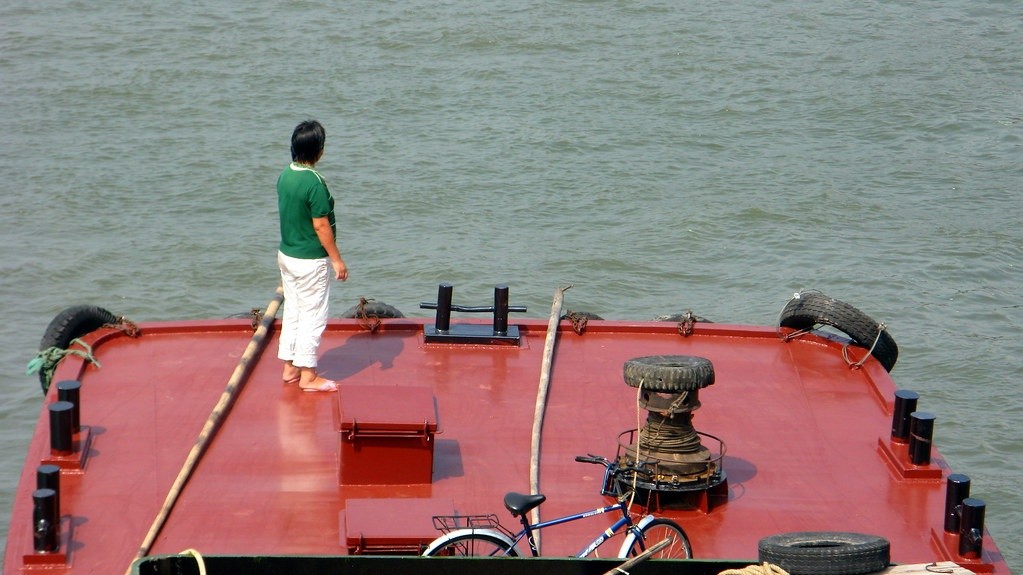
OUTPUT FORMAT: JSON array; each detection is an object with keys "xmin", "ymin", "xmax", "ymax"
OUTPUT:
[
  {"xmin": 757, "ymin": 528, "xmax": 891, "ymax": 575},
  {"xmin": 622, "ymin": 355, "xmax": 717, "ymax": 391},
  {"xmin": 35, "ymin": 305, "xmax": 123, "ymax": 394},
  {"xmin": 560, "ymin": 312, "xmax": 601, "ymax": 323},
  {"xmin": 653, "ymin": 312, "xmax": 714, "ymax": 322},
  {"xmin": 780, "ymin": 288, "xmax": 899, "ymax": 373},
  {"xmin": 343, "ymin": 300, "xmax": 403, "ymax": 321}
]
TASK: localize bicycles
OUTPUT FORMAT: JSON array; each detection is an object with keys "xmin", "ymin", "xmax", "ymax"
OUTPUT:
[{"xmin": 422, "ymin": 452, "xmax": 693, "ymax": 560}]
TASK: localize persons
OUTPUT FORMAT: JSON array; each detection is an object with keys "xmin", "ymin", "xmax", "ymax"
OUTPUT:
[{"xmin": 277, "ymin": 120, "xmax": 348, "ymax": 391}]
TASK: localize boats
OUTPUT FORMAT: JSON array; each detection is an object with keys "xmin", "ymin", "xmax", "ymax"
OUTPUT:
[{"xmin": 2, "ymin": 283, "xmax": 1012, "ymax": 575}]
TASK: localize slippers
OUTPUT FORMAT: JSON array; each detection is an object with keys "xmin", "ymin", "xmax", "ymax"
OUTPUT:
[
  {"xmin": 302, "ymin": 377, "xmax": 339, "ymax": 392},
  {"xmin": 286, "ymin": 377, "xmax": 301, "ymax": 384}
]
